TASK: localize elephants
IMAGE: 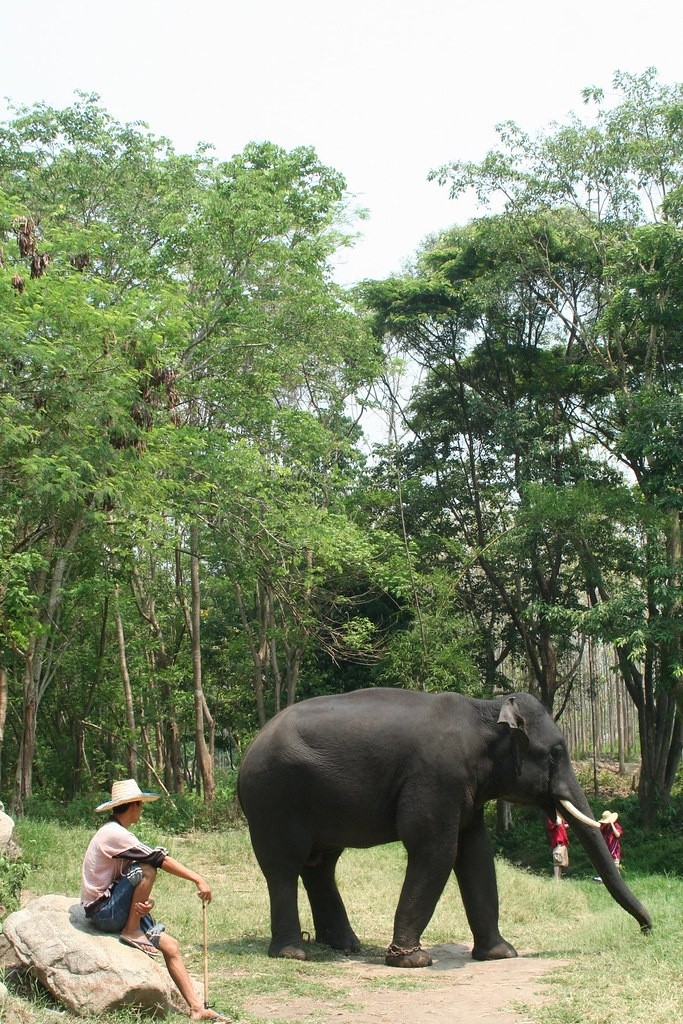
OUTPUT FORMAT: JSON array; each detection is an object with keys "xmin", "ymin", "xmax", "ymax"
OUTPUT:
[{"xmin": 237, "ymin": 687, "xmax": 653, "ymax": 967}]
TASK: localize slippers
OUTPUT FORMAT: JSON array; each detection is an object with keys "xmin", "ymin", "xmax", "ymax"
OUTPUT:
[
  {"xmin": 190, "ymin": 1014, "xmax": 232, "ymax": 1024},
  {"xmin": 120, "ymin": 935, "xmax": 163, "ymax": 957}
]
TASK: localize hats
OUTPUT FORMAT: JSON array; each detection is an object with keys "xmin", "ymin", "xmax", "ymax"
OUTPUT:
[
  {"xmin": 598, "ymin": 810, "xmax": 618, "ymax": 823},
  {"xmin": 95, "ymin": 779, "xmax": 161, "ymax": 813}
]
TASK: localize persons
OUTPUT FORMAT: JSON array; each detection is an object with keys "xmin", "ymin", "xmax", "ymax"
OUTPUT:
[
  {"xmin": 81, "ymin": 778, "xmax": 233, "ymax": 1023},
  {"xmin": 545, "ymin": 808, "xmax": 570, "ymax": 881},
  {"xmin": 593, "ymin": 810, "xmax": 623, "ymax": 883}
]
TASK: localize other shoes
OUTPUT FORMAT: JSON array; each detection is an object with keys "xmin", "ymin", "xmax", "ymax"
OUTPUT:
[{"xmin": 594, "ymin": 876, "xmax": 603, "ymax": 882}]
